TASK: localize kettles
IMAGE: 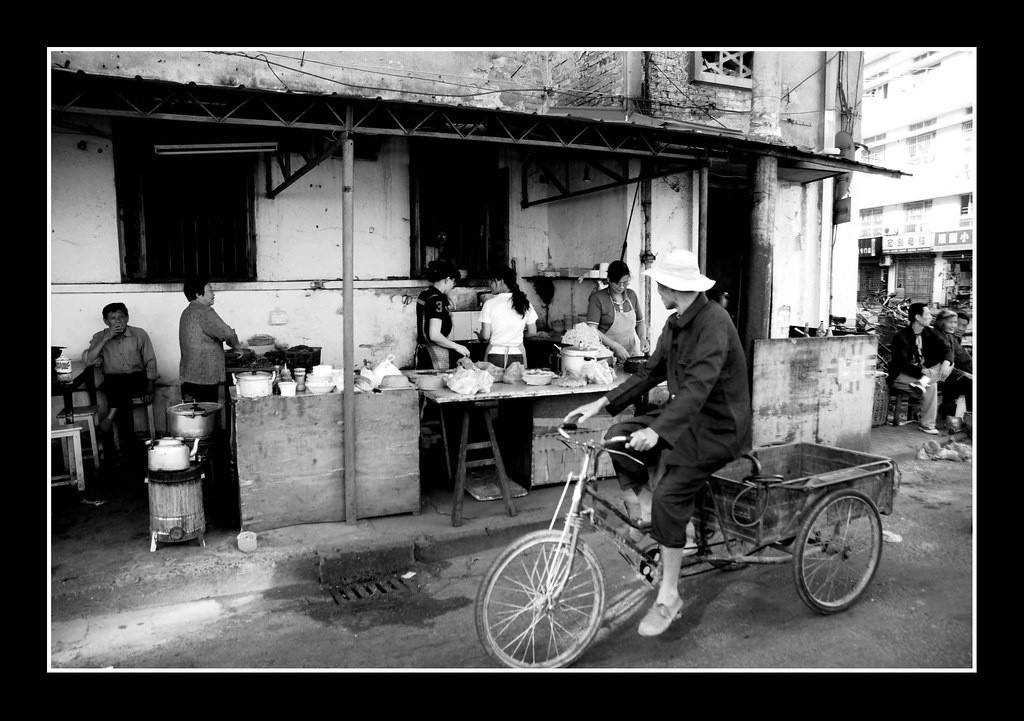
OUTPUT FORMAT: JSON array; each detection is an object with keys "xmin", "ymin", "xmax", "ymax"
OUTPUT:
[{"xmin": 144, "ymin": 435, "xmax": 200, "ymax": 471}]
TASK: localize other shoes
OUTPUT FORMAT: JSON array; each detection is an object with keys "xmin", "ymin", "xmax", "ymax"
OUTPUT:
[
  {"xmin": 100, "ymin": 417, "xmax": 111, "ymax": 431},
  {"xmin": 629, "ymin": 534, "xmax": 657, "ymax": 553},
  {"xmin": 638, "ymin": 596, "xmax": 683, "ymax": 637}
]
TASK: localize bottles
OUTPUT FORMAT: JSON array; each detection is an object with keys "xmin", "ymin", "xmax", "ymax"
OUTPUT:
[
  {"xmin": 802, "ymin": 323, "xmax": 811, "ymax": 338},
  {"xmin": 827, "ymin": 325, "xmax": 833, "ymax": 337},
  {"xmin": 816, "ymin": 321, "xmax": 826, "ymax": 337},
  {"xmin": 281, "ymin": 363, "xmax": 292, "ymax": 382},
  {"xmin": 273, "ymin": 365, "xmax": 282, "ymax": 395},
  {"xmin": 294, "ymin": 368, "xmax": 306, "ymax": 391}
]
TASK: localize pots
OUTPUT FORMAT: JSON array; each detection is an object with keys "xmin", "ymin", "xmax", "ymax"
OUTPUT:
[
  {"xmin": 167, "ymin": 402, "xmax": 223, "ymax": 437},
  {"xmin": 224, "ymin": 348, "xmax": 255, "ymax": 364},
  {"xmin": 232, "ymin": 369, "xmax": 276, "ymax": 397}
]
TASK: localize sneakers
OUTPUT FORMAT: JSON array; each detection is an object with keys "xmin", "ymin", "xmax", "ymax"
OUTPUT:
[
  {"xmin": 908, "ymin": 381, "xmax": 926, "ymax": 396},
  {"xmin": 918, "ymin": 426, "xmax": 940, "ymax": 435}
]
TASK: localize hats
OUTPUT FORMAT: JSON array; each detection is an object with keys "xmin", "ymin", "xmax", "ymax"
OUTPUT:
[{"xmin": 643, "ymin": 248, "xmax": 717, "ymax": 293}]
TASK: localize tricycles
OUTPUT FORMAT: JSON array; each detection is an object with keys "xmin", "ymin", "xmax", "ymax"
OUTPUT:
[{"xmin": 474, "ymin": 423, "xmax": 904, "ymax": 670}]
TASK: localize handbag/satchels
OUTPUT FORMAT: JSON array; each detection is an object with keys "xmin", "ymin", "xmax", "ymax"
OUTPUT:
[{"xmin": 375, "ymin": 354, "xmax": 403, "ymax": 389}]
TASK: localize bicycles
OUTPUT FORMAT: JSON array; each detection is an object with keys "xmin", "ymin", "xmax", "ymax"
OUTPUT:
[{"xmin": 828, "ymin": 289, "xmax": 973, "ymax": 417}]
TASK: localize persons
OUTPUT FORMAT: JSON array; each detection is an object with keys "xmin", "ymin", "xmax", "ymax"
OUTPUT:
[
  {"xmin": 886, "ymin": 303, "xmax": 973, "ymax": 435},
  {"xmin": 564, "ymin": 250, "xmax": 753, "ymax": 636},
  {"xmin": 588, "ymin": 263, "xmax": 650, "ymax": 361},
  {"xmin": 81, "ymin": 302, "xmax": 161, "ymax": 454},
  {"xmin": 416, "ymin": 259, "xmax": 470, "ymax": 439},
  {"xmin": 474, "ymin": 267, "xmax": 538, "ymax": 369},
  {"xmin": 179, "ymin": 277, "xmax": 239, "ymax": 446},
  {"xmin": 888, "ymin": 283, "xmax": 905, "ymax": 309}
]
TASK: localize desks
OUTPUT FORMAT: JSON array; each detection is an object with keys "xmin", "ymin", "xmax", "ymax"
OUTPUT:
[{"xmin": 50, "ymin": 360, "xmax": 100, "ymax": 423}]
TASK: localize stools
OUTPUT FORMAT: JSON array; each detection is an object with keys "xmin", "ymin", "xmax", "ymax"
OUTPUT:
[
  {"xmin": 59, "ymin": 405, "xmax": 102, "ymax": 473},
  {"xmin": 886, "ymin": 380, "xmax": 943, "ymax": 429},
  {"xmin": 108, "ymin": 395, "xmax": 158, "ymax": 453},
  {"xmin": 49, "ymin": 424, "xmax": 86, "ymax": 492}
]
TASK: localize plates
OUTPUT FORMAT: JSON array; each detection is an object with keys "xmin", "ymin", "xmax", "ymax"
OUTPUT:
[{"xmin": 306, "ymin": 366, "xmax": 333, "ymax": 382}]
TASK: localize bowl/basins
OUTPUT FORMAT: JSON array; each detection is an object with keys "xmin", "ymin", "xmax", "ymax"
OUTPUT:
[
  {"xmin": 522, "ymin": 371, "xmax": 554, "ymax": 385},
  {"xmin": 247, "ymin": 338, "xmax": 273, "ymax": 345},
  {"xmin": 305, "ymin": 382, "xmax": 335, "ymax": 395},
  {"xmin": 279, "ymin": 382, "xmax": 297, "ymax": 396}
]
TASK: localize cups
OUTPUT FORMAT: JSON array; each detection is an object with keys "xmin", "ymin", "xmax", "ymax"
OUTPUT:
[
  {"xmin": 237, "ymin": 531, "xmax": 257, "ymax": 552},
  {"xmin": 55, "ymin": 354, "xmax": 72, "ymax": 373}
]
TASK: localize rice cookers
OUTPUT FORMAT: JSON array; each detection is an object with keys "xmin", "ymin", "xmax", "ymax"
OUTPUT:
[{"xmin": 556, "ymin": 342, "xmax": 599, "ymax": 376}]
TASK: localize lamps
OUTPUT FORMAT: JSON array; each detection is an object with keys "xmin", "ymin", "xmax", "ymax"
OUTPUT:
[{"xmin": 152, "ymin": 132, "xmax": 279, "ymax": 156}]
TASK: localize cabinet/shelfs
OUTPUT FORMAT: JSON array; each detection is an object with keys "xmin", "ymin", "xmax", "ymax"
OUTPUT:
[
  {"xmin": 522, "ymin": 265, "xmax": 608, "ymax": 360},
  {"xmin": 403, "ymin": 370, "xmax": 652, "ymax": 530},
  {"xmin": 228, "ymin": 368, "xmax": 425, "ymax": 536}
]
